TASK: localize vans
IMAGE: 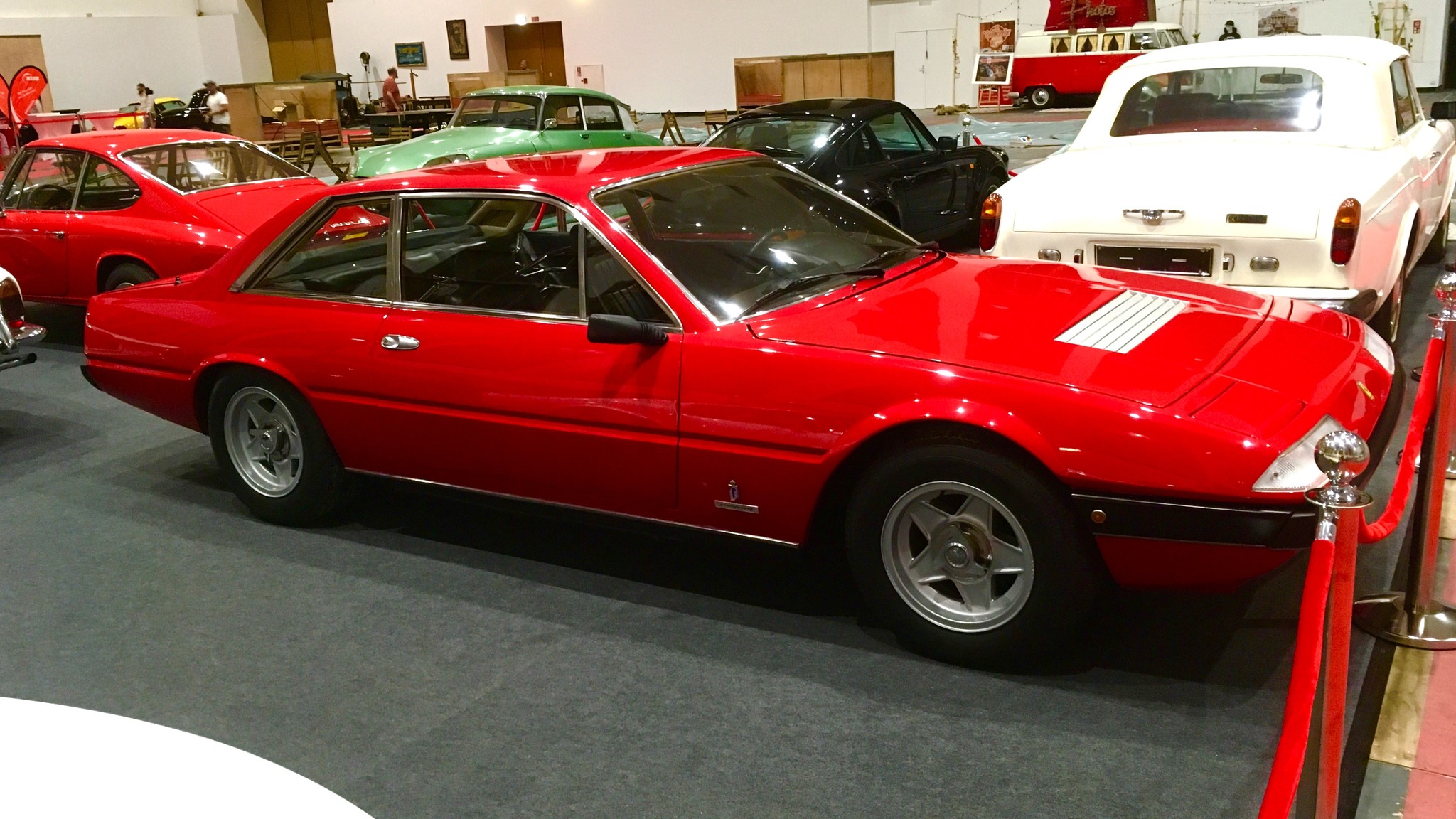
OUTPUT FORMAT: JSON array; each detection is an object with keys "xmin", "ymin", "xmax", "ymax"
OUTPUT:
[{"xmin": 1010, "ymin": 22, "xmax": 1204, "ymax": 110}]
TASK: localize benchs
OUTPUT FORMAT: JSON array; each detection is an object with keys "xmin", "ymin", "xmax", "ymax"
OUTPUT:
[{"xmin": 319, "ymin": 231, "xmax": 527, "ymax": 311}]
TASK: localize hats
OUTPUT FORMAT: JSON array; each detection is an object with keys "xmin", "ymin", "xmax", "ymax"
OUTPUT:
[{"xmin": 203, "ymin": 81, "xmax": 216, "ymax": 86}]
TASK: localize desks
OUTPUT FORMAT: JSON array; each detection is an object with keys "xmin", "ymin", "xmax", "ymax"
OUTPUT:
[
  {"xmin": 374, "ymin": 137, "xmax": 402, "ymax": 146},
  {"xmin": 364, "ymin": 108, "xmax": 456, "ymax": 138},
  {"xmin": 255, "ymin": 139, "xmax": 293, "ymax": 180}
]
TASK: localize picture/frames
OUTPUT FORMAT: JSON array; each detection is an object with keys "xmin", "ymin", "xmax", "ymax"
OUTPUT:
[{"xmin": 395, "ymin": 42, "xmax": 426, "ymax": 66}]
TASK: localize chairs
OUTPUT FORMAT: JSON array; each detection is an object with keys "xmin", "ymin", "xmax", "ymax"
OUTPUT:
[
  {"xmin": 587, "ymin": 251, "xmax": 665, "ymax": 325},
  {"xmin": 110, "ymin": 109, "xmax": 412, "ymax": 191}
]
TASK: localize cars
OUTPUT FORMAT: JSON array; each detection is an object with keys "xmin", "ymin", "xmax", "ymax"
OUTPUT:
[
  {"xmin": 0, "ymin": 128, "xmax": 391, "ymax": 312},
  {"xmin": 0, "ymin": 267, "xmax": 47, "ymax": 371},
  {"xmin": 112, "ymin": 97, "xmax": 188, "ymax": 129},
  {"xmin": 649, "ymin": 96, "xmax": 1010, "ymax": 275},
  {"xmin": 88, "ymin": 148, "xmax": 1409, "ymax": 670},
  {"xmin": 156, "ymin": 87, "xmax": 225, "ymax": 132},
  {"xmin": 344, "ymin": 84, "xmax": 665, "ymax": 228},
  {"xmin": 975, "ymin": 31, "xmax": 1456, "ymax": 346}
]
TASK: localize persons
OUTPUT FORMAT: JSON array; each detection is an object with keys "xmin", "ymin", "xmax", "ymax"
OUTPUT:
[
  {"xmin": 136, "ymin": 83, "xmax": 156, "ymax": 130},
  {"xmin": 203, "ymin": 79, "xmax": 232, "ymax": 137},
  {"xmin": 1218, "ymin": 20, "xmax": 1241, "ymax": 101},
  {"xmin": 382, "ymin": 66, "xmax": 402, "ymax": 111}
]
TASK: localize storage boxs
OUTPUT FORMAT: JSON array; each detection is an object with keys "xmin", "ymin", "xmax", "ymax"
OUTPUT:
[{"xmin": 272, "ymin": 100, "xmax": 299, "ymax": 122}]
